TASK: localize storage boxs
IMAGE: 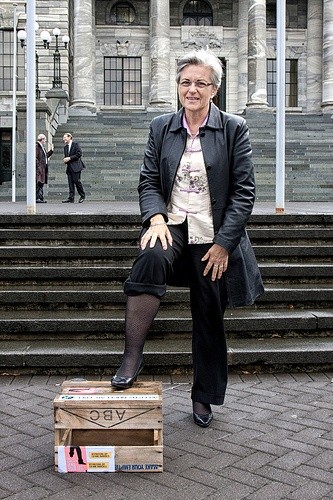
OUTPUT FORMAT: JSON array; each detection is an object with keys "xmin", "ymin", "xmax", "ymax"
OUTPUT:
[{"xmin": 53, "ymin": 379, "xmax": 165, "ymax": 474}]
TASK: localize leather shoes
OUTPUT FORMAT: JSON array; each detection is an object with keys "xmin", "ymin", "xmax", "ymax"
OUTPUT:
[
  {"xmin": 79, "ymin": 197, "xmax": 84, "ymax": 203},
  {"xmin": 61, "ymin": 198, "xmax": 74, "ymax": 203}
]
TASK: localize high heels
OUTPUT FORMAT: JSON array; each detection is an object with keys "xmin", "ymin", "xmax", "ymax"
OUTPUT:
[
  {"xmin": 192, "ymin": 399, "xmax": 213, "ymax": 427},
  {"xmin": 110, "ymin": 358, "xmax": 146, "ymax": 389}
]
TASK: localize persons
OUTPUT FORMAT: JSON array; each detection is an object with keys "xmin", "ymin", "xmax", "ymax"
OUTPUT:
[
  {"xmin": 68, "ymin": 446, "xmax": 86, "ymax": 464},
  {"xmin": 62, "ymin": 133, "xmax": 87, "ymax": 203},
  {"xmin": 108, "ymin": 52, "xmax": 264, "ymax": 428},
  {"xmin": 35, "ymin": 134, "xmax": 55, "ymax": 203}
]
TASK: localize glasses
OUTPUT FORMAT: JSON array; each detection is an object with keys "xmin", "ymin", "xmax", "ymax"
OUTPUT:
[{"xmin": 178, "ymin": 80, "xmax": 213, "ymax": 88}]
